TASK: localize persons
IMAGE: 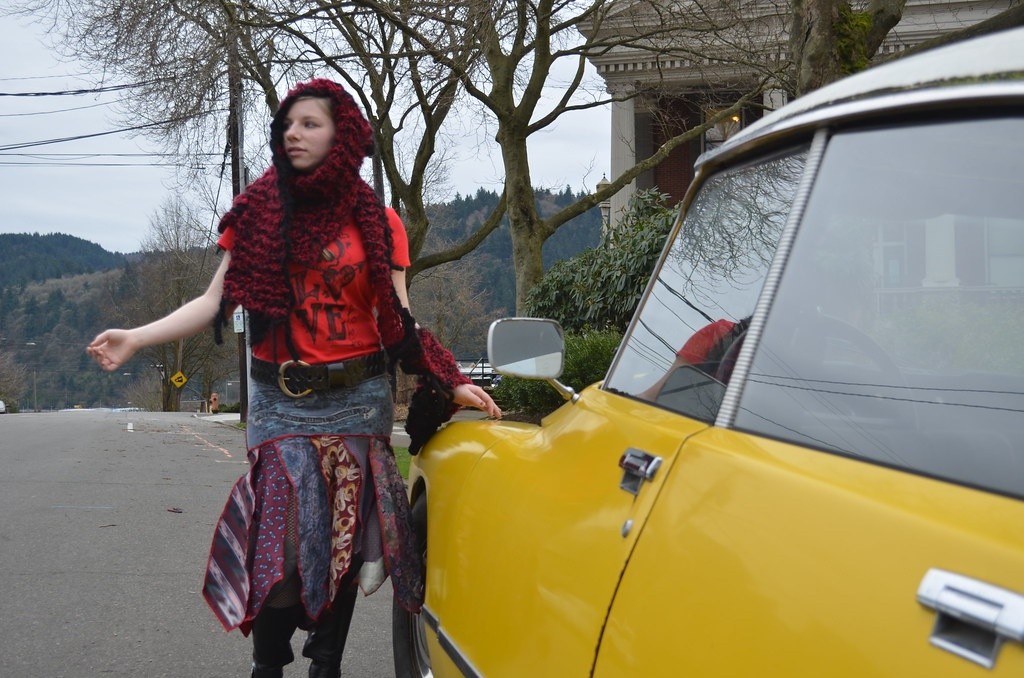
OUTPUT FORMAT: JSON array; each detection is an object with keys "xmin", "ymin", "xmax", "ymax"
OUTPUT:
[
  {"xmin": 84, "ymin": 79, "xmax": 500, "ymax": 678},
  {"xmin": 636, "ymin": 316, "xmax": 753, "ymax": 404}
]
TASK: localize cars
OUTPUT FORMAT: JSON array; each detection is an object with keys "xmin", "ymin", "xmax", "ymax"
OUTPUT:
[{"xmin": 392, "ymin": 28, "xmax": 1024, "ymax": 678}]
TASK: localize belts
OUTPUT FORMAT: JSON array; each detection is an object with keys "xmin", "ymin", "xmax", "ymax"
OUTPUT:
[{"xmin": 250, "ymin": 350, "xmax": 387, "ymax": 397}]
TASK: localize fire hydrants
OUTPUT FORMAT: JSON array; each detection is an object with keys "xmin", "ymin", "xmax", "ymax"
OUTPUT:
[{"xmin": 208, "ymin": 393, "xmax": 219, "ymax": 414}]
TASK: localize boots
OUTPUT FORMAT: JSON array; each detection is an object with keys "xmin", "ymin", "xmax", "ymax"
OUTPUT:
[
  {"xmin": 251, "ymin": 603, "xmax": 305, "ymax": 678},
  {"xmin": 302, "ymin": 603, "xmax": 361, "ymax": 678}
]
